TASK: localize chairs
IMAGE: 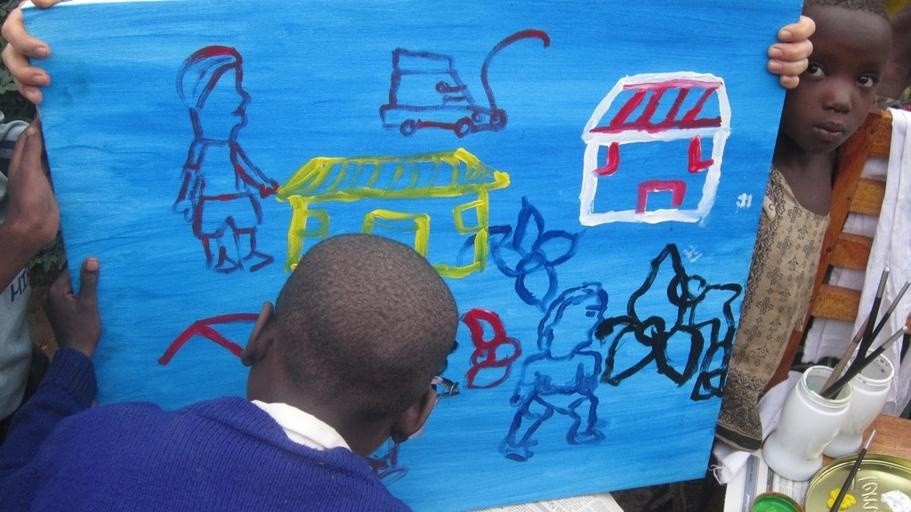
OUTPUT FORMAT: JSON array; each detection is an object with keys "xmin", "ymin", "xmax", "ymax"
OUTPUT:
[{"xmin": 754, "ymin": 106, "xmax": 911, "ymax": 490}]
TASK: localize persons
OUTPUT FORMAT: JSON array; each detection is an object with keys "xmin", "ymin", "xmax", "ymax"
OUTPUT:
[
  {"xmin": 0, "ymin": 173, "xmax": 50, "ymax": 449},
  {"xmin": 1, "ymin": 231, "xmax": 460, "ymax": 511},
  {"xmin": 702, "ymin": 0, "xmax": 893, "ymax": 511},
  {"xmin": 0, "ymin": 116, "xmax": 60, "ymax": 299},
  {"xmin": 2, "ymin": 0, "xmax": 817, "ymax": 108}
]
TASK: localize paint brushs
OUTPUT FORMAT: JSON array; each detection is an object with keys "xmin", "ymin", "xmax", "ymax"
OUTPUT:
[
  {"xmin": 830, "ymin": 430, "xmax": 876, "ymax": 512},
  {"xmin": 820, "ymin": 266, "xmax": 911, "ymax": 400}
]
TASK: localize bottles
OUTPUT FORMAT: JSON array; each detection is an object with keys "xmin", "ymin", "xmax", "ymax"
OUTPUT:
[
  {"xmin": 821, "ymin": 344, "xmax": 895, "ymax": 460},
  {"xmin": 761, "ymin": 365, "xmax": 853, "ymax": 484}
]
